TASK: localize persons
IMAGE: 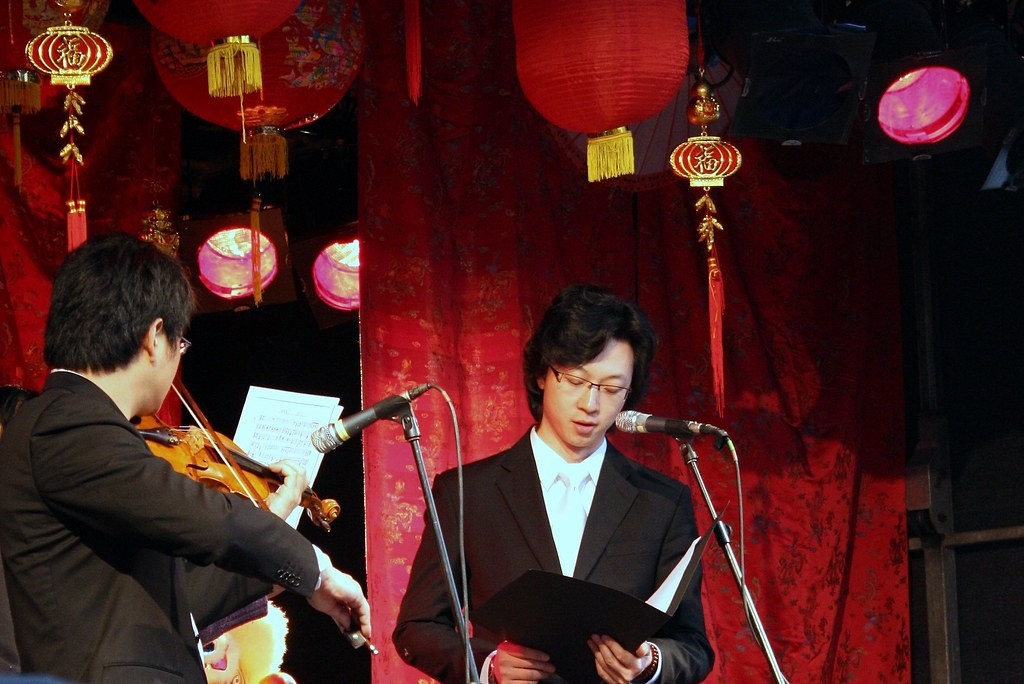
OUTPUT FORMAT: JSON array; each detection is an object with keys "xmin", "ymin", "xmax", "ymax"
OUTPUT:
[
  {"xmin": 0, "ymin": 231, "xmax": 372, "ymax": 684},
  {"xmin": 391, "ymin": 280, "xmax": 717, "ymax": 684}
]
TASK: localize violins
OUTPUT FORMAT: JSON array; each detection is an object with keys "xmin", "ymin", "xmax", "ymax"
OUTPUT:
[{"xmin": 132, "ymin": 410, "xmax": 343, "ymax": 536}]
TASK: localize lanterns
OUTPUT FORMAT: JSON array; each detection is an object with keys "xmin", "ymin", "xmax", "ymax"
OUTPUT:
[
  {"xmin": 134, "ymin": 0, "xmax": 366, "ymax": 308},
  {"xmin": 512, "ymin": 0, "xmax": 691, "ymax": 182},
  {"xmin": 0, "ymin": 0, "xmax": 109, "ymax": 194}
]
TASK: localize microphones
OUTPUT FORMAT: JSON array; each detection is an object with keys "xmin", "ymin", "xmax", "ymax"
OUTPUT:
[
  {"xmin": 311, "ymin": 384, "xmax": 431, "ymax": 453},
  {"xmin": 615, "ymin": 410, "xmax": 727, "ymax": 439}
]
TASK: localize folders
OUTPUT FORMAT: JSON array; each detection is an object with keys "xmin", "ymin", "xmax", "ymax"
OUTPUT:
[{"xmin": 468, "ymin": 499, "xmax": 732, "ymax": 684}]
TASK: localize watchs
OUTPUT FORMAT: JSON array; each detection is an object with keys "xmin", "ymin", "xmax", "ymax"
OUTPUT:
[{"xmin": 488, "ymin": 661, "xmax": 495, "ymax": 684}]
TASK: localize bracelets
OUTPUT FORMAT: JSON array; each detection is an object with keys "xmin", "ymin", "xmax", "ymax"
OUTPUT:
[{"xmin": 634, "ymin": 645, "xmax": 658, "ymax": 680}]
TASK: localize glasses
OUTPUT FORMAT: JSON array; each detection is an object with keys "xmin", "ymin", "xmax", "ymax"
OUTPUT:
[
  {"xmin": 170, "ymin": 330, "xmax": 192, "ymax": 356},
  {"xmin": 548, "ymin": 364, "xmax": 632, "ymax": 403}
]
